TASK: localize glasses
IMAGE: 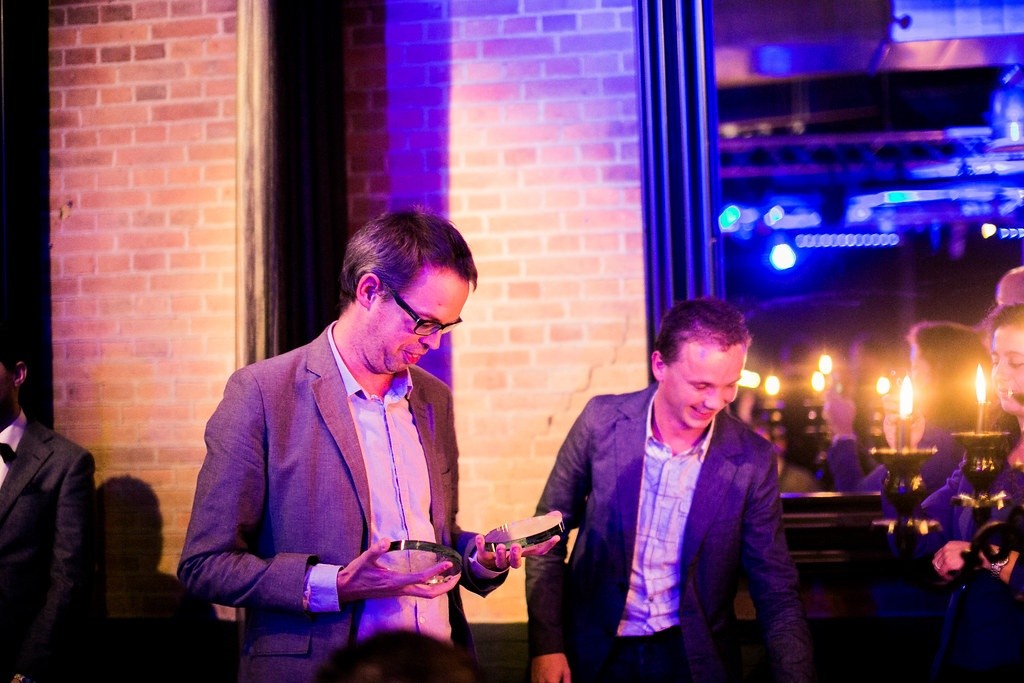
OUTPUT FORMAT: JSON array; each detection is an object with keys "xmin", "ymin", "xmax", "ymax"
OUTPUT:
[{"xmin": 382, "ymin": 280, "xmax": 463, "ymax": 336}]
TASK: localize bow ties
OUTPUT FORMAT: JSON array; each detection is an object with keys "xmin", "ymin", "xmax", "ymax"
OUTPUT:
[{"xmin": 0, "ymin": 443, "xmax": 18, "ymax": 462}]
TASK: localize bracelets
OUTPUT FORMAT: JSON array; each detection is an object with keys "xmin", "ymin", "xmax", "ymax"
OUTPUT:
[{"xmin": 13, "ymin": 674, "xmax": 31, "ymax": 683}]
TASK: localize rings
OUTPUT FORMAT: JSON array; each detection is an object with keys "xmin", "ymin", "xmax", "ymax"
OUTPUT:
[{"xmin": 934, "ymin": 561, "xmax": 941, "ymax": 569}]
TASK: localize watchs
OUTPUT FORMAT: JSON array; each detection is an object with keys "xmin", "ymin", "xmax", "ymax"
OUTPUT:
[{"xmin": 990, "ymin": 546, "xmax": 1010, "ymax": 578}]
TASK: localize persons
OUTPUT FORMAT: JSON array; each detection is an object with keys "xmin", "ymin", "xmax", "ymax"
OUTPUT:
[
  {"xmin": 310, "ymin": 628, "xmax": 485, "ymax": 683},
  {"xmin": 823, "ymin": 322, "xmax": 995, "ymax": 494},
  {"xmin": 752, "ymin": 418, "xmax": 816, "ymax": 491},
  {"xmin": 177, "ymin": 212, "xmax": 562, "ymax": 683},
  {"xmin": 524, "ymin": 299, "xmax": 815, "ymax": 683},
  {"xmin": 0, "ymin": 329, "xmax": 96, "ymax": 683},
  {"xmin": 882, "ymin": 301, "xmax": 1024, "ymax": 683}
]
